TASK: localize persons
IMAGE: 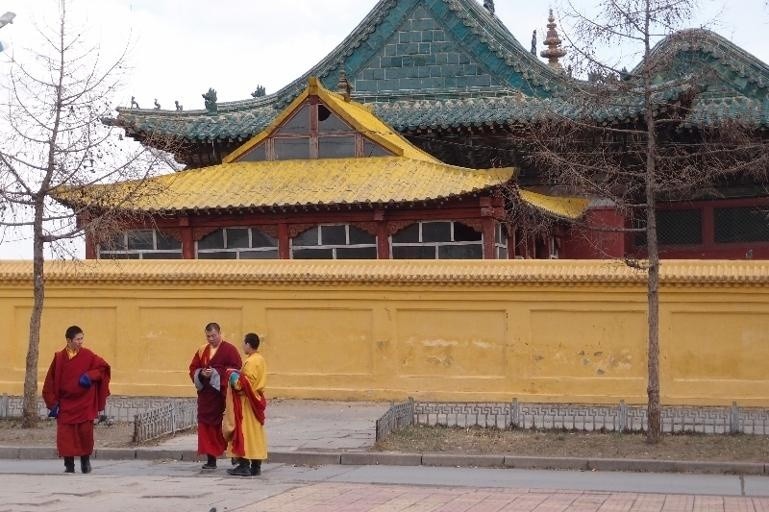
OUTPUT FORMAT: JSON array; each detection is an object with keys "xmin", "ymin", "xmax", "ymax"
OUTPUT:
[
  {"xmin": 190, "ymin": 323, "xmax": 242, "ymax": 469},
  {"xmin": 41, "ymin": 325, "xmax": 112, "ymax": 474},
  {"xmin": 222, "ymin": 332, "xmax": 268, "ymax": 475}
]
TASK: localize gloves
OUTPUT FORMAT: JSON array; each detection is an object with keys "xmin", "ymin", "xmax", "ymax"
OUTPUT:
[
  {"xmin": 79, "ymin": 374, "xmax": 92, "ymax": 386},
  {"xmin": 48, "ymin": 404, "xmax": 60, "ymax": 418}
]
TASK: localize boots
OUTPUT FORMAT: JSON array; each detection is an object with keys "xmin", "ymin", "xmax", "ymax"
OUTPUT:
[
  {"xmin": 80, "ymin": 455, "xmax": 91, "ymax": 473},
  {"xmin": 202, "ymin": 454, "xmax": 217, "ymax": 469},
  {"xmin": 227, "ymin": 458, "xmax": 262, "ymax": 476},
  {"xmin": 65, "ymin": 455, "xmax": 74, "ymax": 474}
]
